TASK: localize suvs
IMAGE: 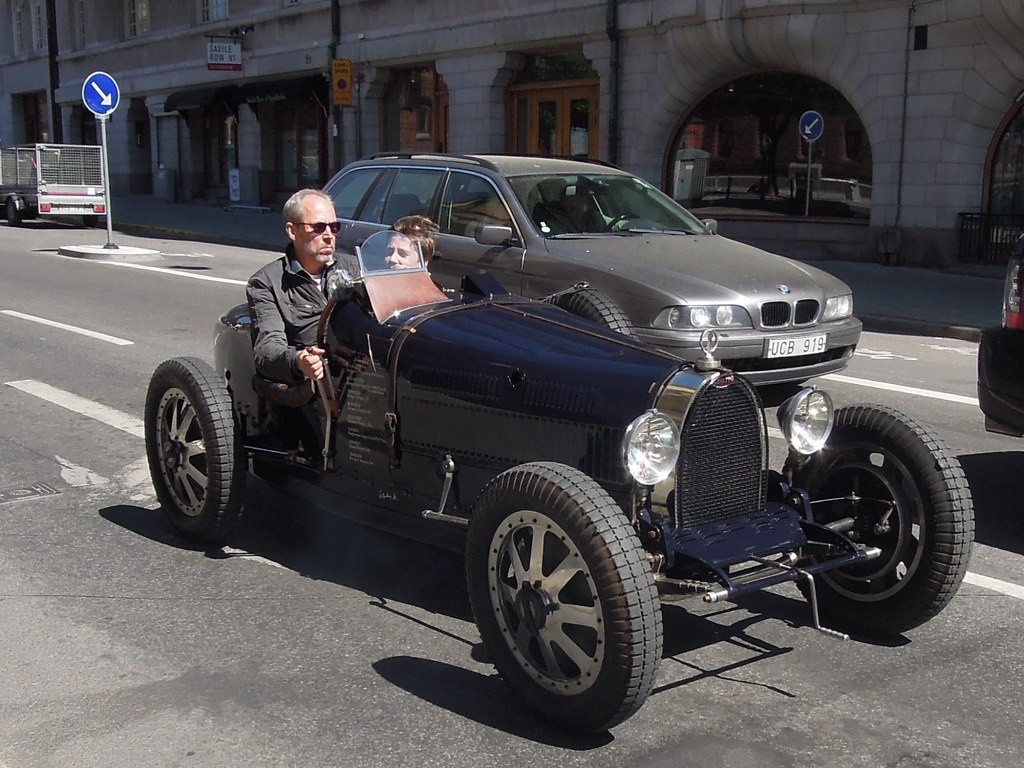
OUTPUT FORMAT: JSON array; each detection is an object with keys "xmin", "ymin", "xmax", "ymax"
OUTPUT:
[{"xmin": 321, "ymin": 151, "xmax": 862, "ymax": 387}]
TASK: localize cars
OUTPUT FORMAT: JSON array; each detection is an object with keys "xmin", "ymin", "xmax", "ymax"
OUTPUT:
[
  {"xmin": 977, "ymin": 236, "xmax": 1024, "ymax": 437},
  {"xmin": 145, "ymin": 229, "xmax": 974, "ymax": 740}
]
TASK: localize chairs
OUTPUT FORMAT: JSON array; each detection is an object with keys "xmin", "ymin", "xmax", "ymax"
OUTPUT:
[
  {"xmin": 451, "ymin": 191, "xmax": 487, "ymax": 237},
  {"xmin": 531, "ymin": 200, "xmax": 558, "ymax": 226}
]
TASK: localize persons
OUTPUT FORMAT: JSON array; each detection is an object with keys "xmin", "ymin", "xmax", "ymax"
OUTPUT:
[
  {"xmin": 384, "ymin": 215, "xmax": 440, "ymax": 269},
  {"xmin": 246, "ymin": 189, "xmax": 369, "ymax": 464}
]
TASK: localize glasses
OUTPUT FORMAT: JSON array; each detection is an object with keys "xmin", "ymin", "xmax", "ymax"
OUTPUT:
[{"xmin": 293, "ymin": 222, "xmax": 343, "ymax": 235}]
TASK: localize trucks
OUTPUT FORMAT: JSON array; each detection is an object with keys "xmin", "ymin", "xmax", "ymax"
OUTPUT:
[{"xmin": 0, "ymin": 142, "xmax": 107, "ymax": 227}]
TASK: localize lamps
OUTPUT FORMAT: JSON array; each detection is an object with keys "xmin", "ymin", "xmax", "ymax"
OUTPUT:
[{"xmin": 241, "ymin": 24, "xmax": 255, "ymax": 35}]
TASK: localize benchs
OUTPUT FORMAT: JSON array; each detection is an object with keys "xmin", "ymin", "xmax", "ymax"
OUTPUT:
[{"xmin": 384, "ymin": 195, "xmax": 434, "ymax": 227}]
